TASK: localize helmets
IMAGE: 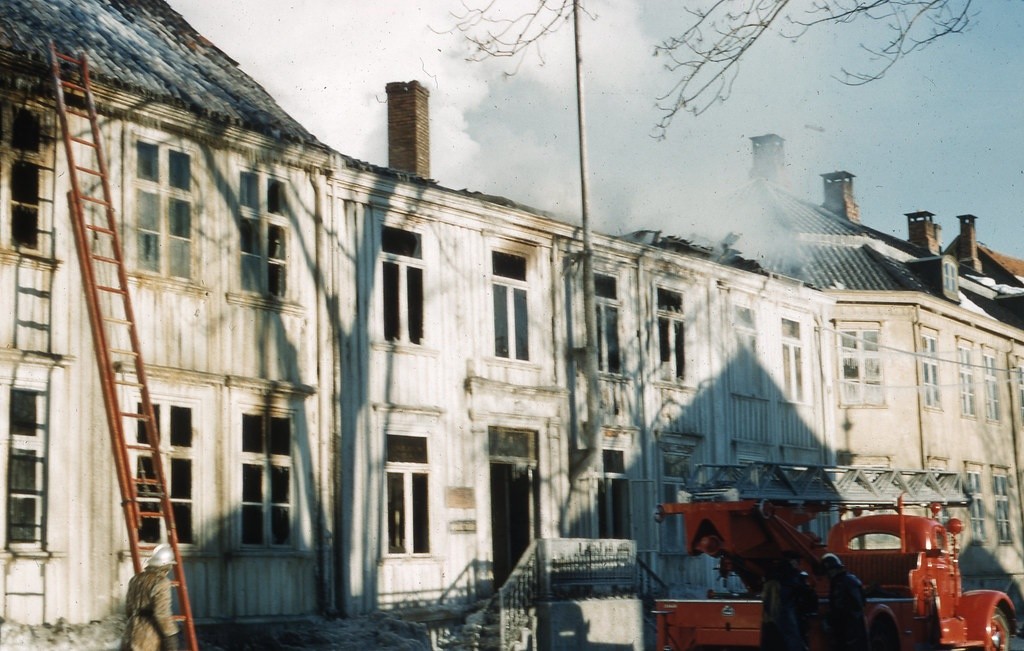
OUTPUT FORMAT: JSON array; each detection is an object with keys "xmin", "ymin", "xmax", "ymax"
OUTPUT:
[{"xmin": 146, "ymin": 543, "xmax": 178, "ymax": 567}]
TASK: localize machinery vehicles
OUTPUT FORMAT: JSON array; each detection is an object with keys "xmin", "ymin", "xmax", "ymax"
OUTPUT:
[{"xmin": 648, "ymin": 461, "xmax": 1018, "ymax": 650}]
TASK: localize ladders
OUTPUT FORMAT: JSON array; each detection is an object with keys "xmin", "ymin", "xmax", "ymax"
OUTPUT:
[{"xmin": 44, "ymin": 36, "xmax": 197, "ymax": 651}]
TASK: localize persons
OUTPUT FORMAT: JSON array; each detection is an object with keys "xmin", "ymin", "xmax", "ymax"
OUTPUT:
[
  {"xmin": 760, "ymin": 551, "xmax": 817, "ymax": 651},
  {"xmin": 122, "ymin": 545, "xmax": 181, "ymax": 651},
  {"xmin": 818, "ymin": 553, "xmax": 872, "ymax": 651}
]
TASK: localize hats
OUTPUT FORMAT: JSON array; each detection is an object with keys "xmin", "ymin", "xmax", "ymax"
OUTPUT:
[
  {"xmin": 778, "ymin": 551, "xmax": 803, "ymax": 568},
  {"xmin": 821, "ymin": 553, "xmax": 842, "ymax": 565}
]
